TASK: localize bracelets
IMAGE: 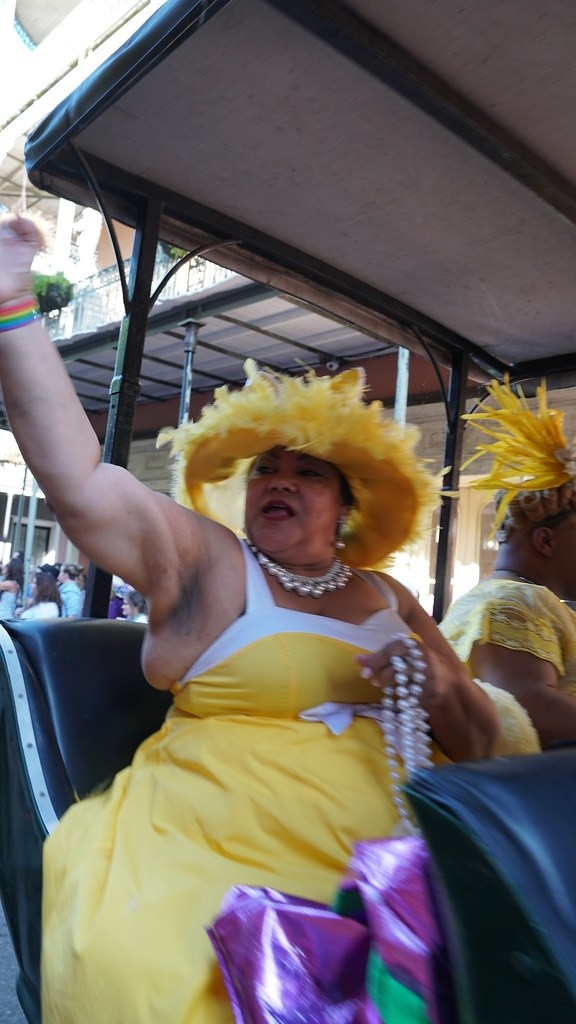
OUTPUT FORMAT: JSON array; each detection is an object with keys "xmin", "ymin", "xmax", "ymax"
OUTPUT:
[{"xmin": 0, "ymin": 298, "xmax": 42, "ymax": 333}]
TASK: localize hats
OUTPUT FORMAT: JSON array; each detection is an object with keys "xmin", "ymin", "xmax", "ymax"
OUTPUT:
[
  {"xmin": 155, "ymin": 357, "xmax": 451, "ymax": 569},
  {"xmin": 38, "ymin": 564, "xmax": 59, "ymax": 581}
]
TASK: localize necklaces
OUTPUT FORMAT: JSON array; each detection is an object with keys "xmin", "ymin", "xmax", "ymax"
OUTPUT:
[
  {"xmin": 495, "ymin": 568, "xmax": 539, "ymax": 586},
  {"xmin": 244, "ymin": 537, "xmax": 354, "ymax": 597},
  {"xmin": 359, "ymin": 633, "xmax": 438, "ymax": 844}
]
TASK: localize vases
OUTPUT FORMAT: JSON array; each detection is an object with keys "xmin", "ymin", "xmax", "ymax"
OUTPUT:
[{"xmin": 31, "ymin": 270, "xmax": 74, "ymax": 310}]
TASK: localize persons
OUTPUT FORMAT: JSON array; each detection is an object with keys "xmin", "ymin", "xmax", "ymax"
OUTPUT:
[
  {"xmin": 0, "ymin": 551, "xmax": 149, "ymax": 625},
  {"xmin": 436, "ymin": 395, "xmax": 576, "ymax": 754},
  {"xmin": 0, "ymin": 220, "xmax": 541, "ymax": 1024}
]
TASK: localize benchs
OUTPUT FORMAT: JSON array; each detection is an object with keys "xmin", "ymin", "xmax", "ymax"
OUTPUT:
[{"xmin": 0, "ymin": 615, "xmax": 576, "ymax": 1022}]
